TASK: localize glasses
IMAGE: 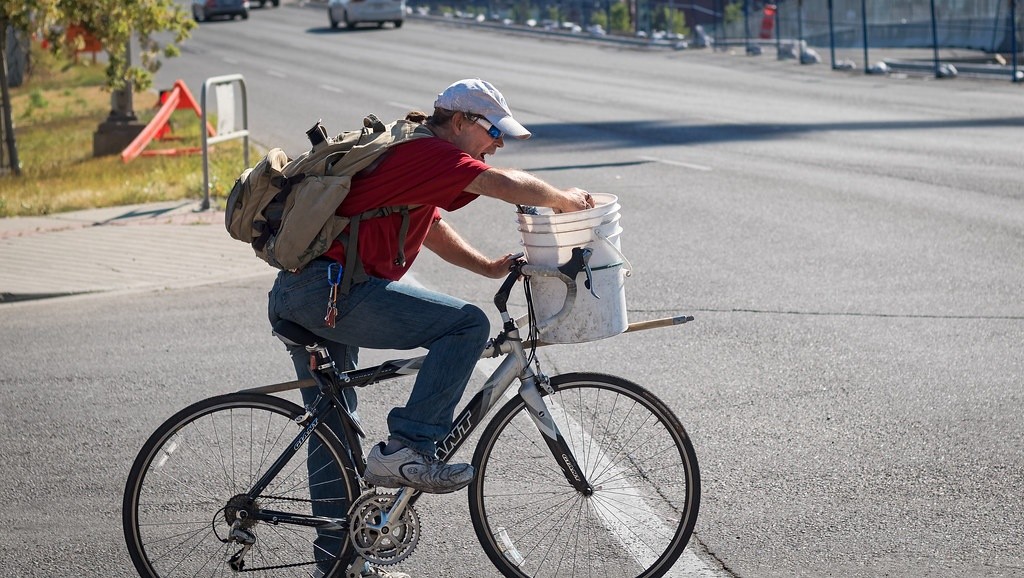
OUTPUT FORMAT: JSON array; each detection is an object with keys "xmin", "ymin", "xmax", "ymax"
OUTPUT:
[{"xmin": 464, "ymin": 114, "xmax": 505, "ymax": 139}]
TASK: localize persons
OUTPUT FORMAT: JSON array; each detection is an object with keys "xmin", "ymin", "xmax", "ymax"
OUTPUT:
[{"xmin": 268, "ymin": 80, "xmax": 596, "ymax": 578}]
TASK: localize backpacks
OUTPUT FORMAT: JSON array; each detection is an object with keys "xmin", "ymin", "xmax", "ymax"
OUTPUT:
[{"xmin": 224, "ymin": 119, "xmax": 435, "ymax": 276}]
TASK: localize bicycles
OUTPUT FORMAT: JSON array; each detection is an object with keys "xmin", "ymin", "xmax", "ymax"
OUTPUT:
[{"xmin": 120, "ymin": 243, "xmax": 701, "ymax": 577}]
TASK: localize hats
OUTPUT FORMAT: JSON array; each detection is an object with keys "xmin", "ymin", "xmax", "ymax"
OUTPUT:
[{"xmin": 434, "ymin": 76, "xmax": 533, "ymax": 140}]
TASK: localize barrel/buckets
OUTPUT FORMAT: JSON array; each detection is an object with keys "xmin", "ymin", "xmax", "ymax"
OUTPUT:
[{"xmin": 513, "ymin": 193, "xmax": 633, "ymax": 344}]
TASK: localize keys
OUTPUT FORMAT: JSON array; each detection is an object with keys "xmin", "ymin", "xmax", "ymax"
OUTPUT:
[{"xmin": 324, "ymin": 300, "xmax": 338, "ymax": 329}]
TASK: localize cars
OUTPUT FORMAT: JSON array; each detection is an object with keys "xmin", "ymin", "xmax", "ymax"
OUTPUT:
[
  {"xmin": 191, "ymin": 0, "xmax": 250, "ymax": 22},
  {"xmin": 327, "ymin": 0, "xmax": 408, "ymax": 29}
]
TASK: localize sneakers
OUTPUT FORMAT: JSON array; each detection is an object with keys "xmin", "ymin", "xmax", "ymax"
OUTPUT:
[
  {"xmin": 366, "ymin": 444, "xmax": 477, "ymax": 494},
  {"xmin": 313, "ymin": 558, "xmax": 410, "ymax": 578}
]
